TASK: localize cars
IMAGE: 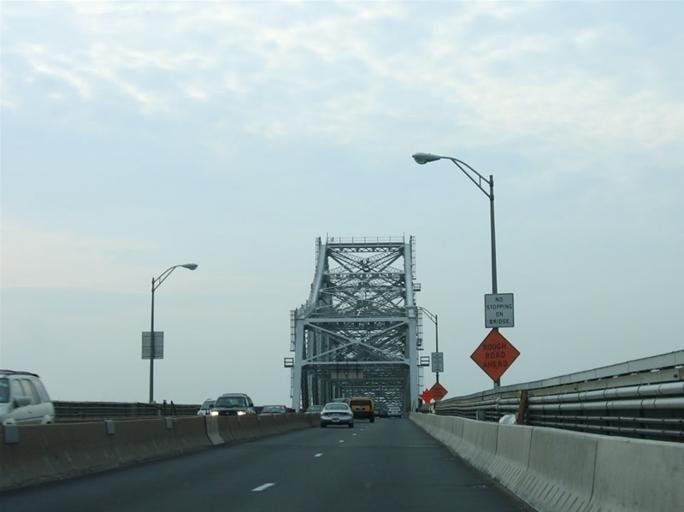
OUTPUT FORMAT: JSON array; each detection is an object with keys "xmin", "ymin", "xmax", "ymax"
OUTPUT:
[
  {"xmin": 258, "ymin": 404, "xmax": 288, "ymax": 416},
  {"xmin": 303, "ymin": 395, "xmax": 402, "ymax": 429}
]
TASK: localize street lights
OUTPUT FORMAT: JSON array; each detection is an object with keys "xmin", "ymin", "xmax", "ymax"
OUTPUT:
[
  {"xmin": 412, "ymin": 151, "xmax": 501, "ymax": 392},
  {"xmin": 405, "ymin": 304, "xmax": 438, "ymax": 414},
  {"xmin": 148, "ymin": 263, "xmax": 198, "ymax": 404}
]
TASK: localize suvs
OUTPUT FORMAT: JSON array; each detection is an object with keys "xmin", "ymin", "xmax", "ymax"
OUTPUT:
[
  {"xmin": 197, "ymin": 397, "xmax": 221, "ymax": 415},
  {"xmin": 209, "ymin": 393, "xmax": 257, "ymax": 416},
  {"xmin": 0, "ymin": 368, "xmax": 56, "ymax": 427}
]
